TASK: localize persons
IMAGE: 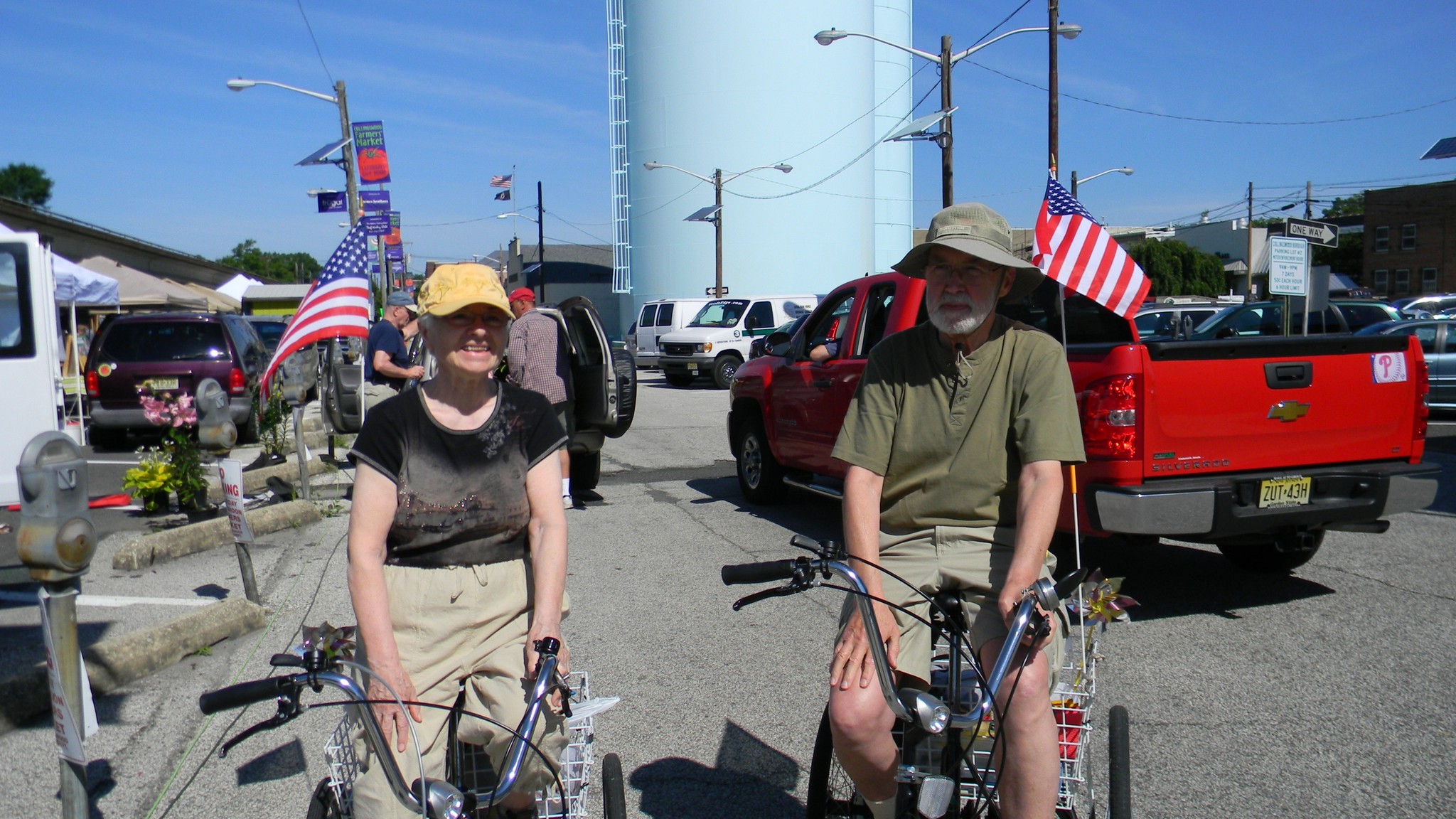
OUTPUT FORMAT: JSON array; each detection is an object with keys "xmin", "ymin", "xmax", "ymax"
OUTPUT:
[
  {"xmin": 344, "ymin": 262, "xmax": 568, "ymax": 818},
  {"xmin": 809, "ymin": 337, "xmax": 843, "ymax": 361},
  {"xmin": 507, "ymin": 287, "xmax": 573, "ymax": 509},
  {"xmin": 826, "ymin": 202, "xmax": 1087, "ymax": 819}
]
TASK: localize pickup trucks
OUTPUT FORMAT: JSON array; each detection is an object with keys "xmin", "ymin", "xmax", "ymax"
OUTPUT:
[{"xmin": 726, "ymin": 270, "xmax": 1432, "ymax": 570}]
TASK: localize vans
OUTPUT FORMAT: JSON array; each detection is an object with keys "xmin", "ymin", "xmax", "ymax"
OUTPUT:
[
  {"xmin": 634, "ymin": 298, "xmax": 722, "ymax": 366},
  {"xmin": 657, "ymin": 292, "xmax": 854, "ymax": 390}
]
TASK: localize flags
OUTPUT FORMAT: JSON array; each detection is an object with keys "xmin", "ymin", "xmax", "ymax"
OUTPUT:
[
  {"xmin": 493, "ymin": 189, "xmax": 511, "ymax": 202},
  {"xmin": 251, "ymin": 216, "xmax": 371, "ymax": 391},
  {"xmin": 1031, "ymin": 170, "xmax": 1153, "ymax": 322},
  {"xmin": 489, "ymin": 174, "xmax": 513, "ymax": 188}
]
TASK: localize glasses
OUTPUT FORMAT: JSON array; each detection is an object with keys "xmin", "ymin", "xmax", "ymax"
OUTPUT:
[
  {"xmin": 433, "ymin": 310, "xmax": 512, "ymax": 328},
  {"xmin": 922, "ymin": 261, "xmax": 1005, "ymax": 286}
]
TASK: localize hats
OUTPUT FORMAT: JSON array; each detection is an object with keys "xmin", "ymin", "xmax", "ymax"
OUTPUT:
[
  {"xmin": 417, "ymin": 262, "xmax": 516, "ymax": 322},
  {"xmin": 889, "ymin": 202, "xmax": 1040, "ymax": 290},
  {"xmin": 386, "ymin": 291, "xmax": 418, "ymax": 313},
  {"xmin": 508, "ymin": 287, "xmax": 535, "ymax": 303}
]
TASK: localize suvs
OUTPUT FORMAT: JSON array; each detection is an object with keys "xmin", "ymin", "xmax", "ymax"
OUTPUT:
[{"xmin": 320, "ymin": 296, "xmax": 638, "ymax": 490}]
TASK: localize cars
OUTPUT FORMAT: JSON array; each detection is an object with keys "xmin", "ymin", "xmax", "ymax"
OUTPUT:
[
  {"xmin": 80, "ymin": 309, "xmax": 277, "ymax": 448},
  {"xmin": 1131, "ymin": 292, "xmax": 1456, "ymax": 421},
  {"xmin": 247, "ymin": 314, "xmax": 319, "ymax": 405}
]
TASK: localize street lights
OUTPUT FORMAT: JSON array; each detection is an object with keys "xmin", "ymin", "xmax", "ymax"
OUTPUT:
[
  {"xmin": 643, "ymin": 161, "xmax": 793, "ymax": 299},
  {"xmin": 497, "ymin": 212, "xmax": 545, "ymax": 303},
  {"xmin": 473, "ymin": 254, "xmax": 503, "ymax": 286},
  {"xmin": 815, "ymin": 20, "xmax": 1083, "ymax": 211},
  {"xmin": 227, "ymin": 78, "xmax": 393, "ymax": 319},
  {"xmin": 1072, "ymin": 167, "xmax": 1134, "ymax": 198}
]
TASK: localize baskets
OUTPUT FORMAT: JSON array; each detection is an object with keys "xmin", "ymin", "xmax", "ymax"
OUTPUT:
[
  {"xmin": 325, "ymin": 670, "xmax": 593, "ymax": 819},
  {"xmin": 927, "ymin": 630, "xmax": 1096, "ymax": 809}
]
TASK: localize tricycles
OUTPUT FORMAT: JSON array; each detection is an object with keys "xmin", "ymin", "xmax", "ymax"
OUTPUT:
[
  {"xmin": 722, "ymin": 535, "xmax": 1133, "ymax": 819},
  {"xmin": 198, "ymin": 635, "xmax": 625, "ymax": 819}
]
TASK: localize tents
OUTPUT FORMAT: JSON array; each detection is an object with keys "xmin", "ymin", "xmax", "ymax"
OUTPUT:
[{"xmin": 0, "ymin": 218, "xmax": 275, "ymax": 446}]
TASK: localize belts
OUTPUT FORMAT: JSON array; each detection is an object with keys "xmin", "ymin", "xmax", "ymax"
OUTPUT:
[{"xmin": 367, "ymin": 378, "xmax": 401, "ymax": 392}]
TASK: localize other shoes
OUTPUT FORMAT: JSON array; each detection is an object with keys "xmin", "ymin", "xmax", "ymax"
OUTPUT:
[{"xmin": 562, "ymin": 494, "xmax": 574, "ymax": 509}]
